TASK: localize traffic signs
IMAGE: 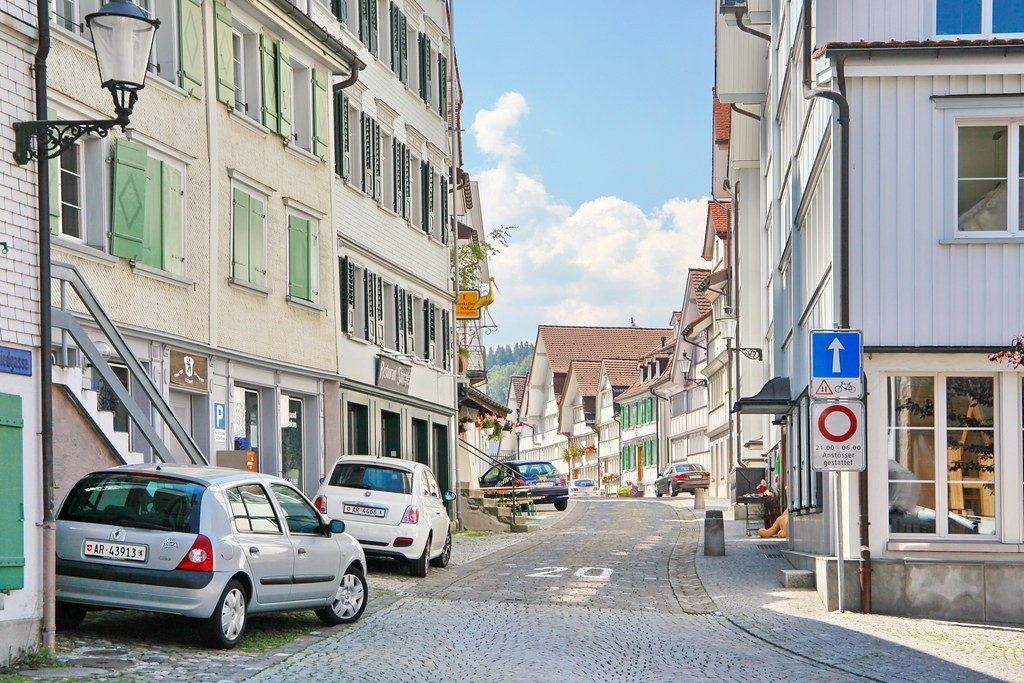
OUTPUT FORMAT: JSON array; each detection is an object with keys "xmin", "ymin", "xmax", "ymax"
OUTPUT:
[{"xmin": 807, "ymin": 329, "xmax": 866, "ymax": 401}]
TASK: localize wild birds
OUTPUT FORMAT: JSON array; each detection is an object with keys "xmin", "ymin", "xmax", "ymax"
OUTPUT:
[{"xmin": 470, "ymin": 277, "xmax": 500, "ymax": 326}]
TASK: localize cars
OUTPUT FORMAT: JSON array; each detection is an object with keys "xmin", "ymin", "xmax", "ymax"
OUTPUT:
[
  {"xmin": 653, "ymin": 462, "xmax": 710, "ymax": 496},
  {"xmin": 478, "ymin": 459, "xmax": 568, "ymax": 511},
  {"xmin": 52, "ymin": 462, "xmax": 369, "ymax": 648},
  {"xmin": 307, "ymin": 454, "xmax": 459, "ymax": 578},
  {"xmin": 567, "ymin": 479, "xmax": 601, "ymax": 497}
]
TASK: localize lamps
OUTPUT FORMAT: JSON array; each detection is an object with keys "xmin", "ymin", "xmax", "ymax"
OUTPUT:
[{"xmin": 12, "ymin": 0, "xmax": 161, "ymax": 165}]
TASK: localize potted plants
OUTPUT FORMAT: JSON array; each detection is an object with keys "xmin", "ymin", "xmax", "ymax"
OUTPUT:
[
  {"xmin": 617, "ymin": 487, "xmax": 630, "ymax": 497},
  {"xmin": 481, "ymin": 419, "xmax": 495, "ymax": 436},
  {"xmin": 459, "ymin": 417, "xmax": 475, "ymax": 429}
]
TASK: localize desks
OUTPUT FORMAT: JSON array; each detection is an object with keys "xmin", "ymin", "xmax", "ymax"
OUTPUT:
[
  {"xmin": 481, "ymin": 484, "xmax": 534, "ymax": 515},
  {"xmin": 737, "ymin": 496, "xmax": 771, "ymax": 537}
]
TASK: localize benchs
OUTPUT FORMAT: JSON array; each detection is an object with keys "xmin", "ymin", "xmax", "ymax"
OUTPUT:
[{"xmin": 492, "ymin": 495, "xmax": 546, "ymax": 516}]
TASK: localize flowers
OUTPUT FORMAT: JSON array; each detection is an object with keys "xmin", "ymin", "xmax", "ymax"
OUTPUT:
[{"xmin": 579, "ymin": 444, "xmax": 597, "ymax": 453}]
